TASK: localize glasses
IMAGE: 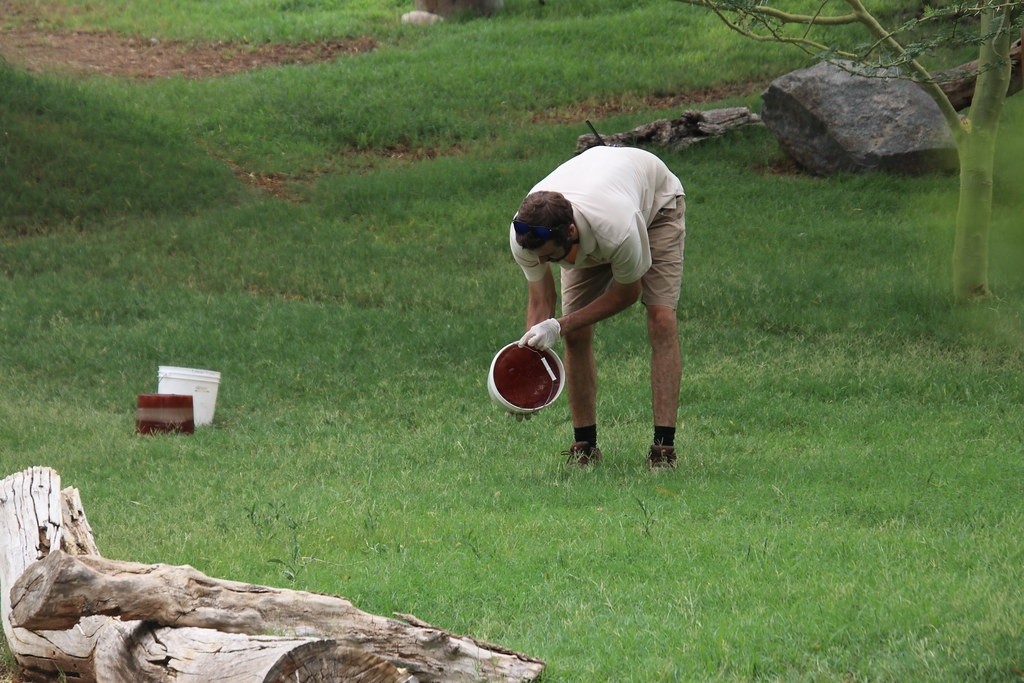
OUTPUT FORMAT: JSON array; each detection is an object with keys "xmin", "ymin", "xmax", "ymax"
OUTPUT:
[{"xmin": 512, "ymin": 211, "xmax": 569, "ymax": 240}]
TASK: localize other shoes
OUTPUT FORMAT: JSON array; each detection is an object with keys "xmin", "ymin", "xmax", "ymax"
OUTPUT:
[
  {"xmin": 560, "ymin": 441, "xmax": 602, "ymax": 469},
  {"xmin": 647, "ymin": 444, "xmax": 678, "ymax": 472}
]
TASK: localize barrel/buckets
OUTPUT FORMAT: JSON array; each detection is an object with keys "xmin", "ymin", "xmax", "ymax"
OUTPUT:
[
  {"xmin": 157, "ymin": 366, "xmax": 221, "ymax": 428},
  {"xmin": 487, "ymin": 341, "xmax": 565, "ymax": 414}
]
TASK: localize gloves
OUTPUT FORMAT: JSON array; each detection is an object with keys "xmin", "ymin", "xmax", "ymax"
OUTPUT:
[
  {"xmin": 518, "ymin": 318, "xmax": 561, "ymax": 350},
  {"xmin": 505, "ymin": 411, "xmax": 539, "ymax": 422}
]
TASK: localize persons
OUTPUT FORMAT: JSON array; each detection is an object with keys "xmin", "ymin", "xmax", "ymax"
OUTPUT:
[{"xmin": 505, "ymin": 146, "xmax": 686, "ymax": 486}]
